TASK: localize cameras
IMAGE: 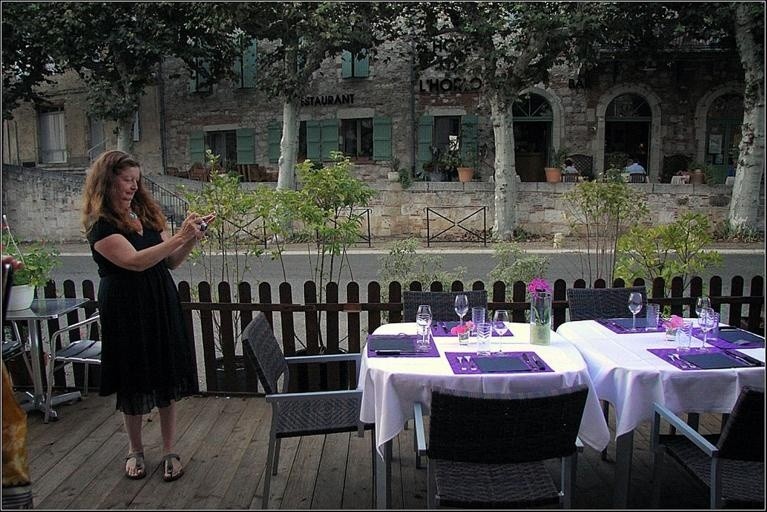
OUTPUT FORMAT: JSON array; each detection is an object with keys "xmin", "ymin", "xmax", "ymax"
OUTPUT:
[{"xmin": 193, "ymin": 216, "xmax": 209, "ymax": 234}]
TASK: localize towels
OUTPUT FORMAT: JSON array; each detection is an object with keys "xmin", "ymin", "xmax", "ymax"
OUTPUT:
[
  {"xmin": 445, "ymin": 351, "xmax": 556, "ymax": 374},
  {"xmin": 647, "ymin": 346, "xmax": 765, "ymax": 370},
  {"xmin": 596, "ymin": 317, "xmax": 670, "ymax": 334},
  {"xmin": 685, "ymin": 326, "xmax": 764, "ymax": 349},
  {"xmin": 430, "ymin": 319, "xmax": 514, "ymax": 337},
  {"xmin": 367, "ymin": 334, "xmax": 440, "ymax": 358}
]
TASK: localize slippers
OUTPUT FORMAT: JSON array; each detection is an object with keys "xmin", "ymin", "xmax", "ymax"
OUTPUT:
[
  {"xmin": 159, "ymin": 453, "xmax": 184, "ymax": 481},
  {"xmin": 122, "ymin": 451, "xmax": 146, "ymax": 479}
]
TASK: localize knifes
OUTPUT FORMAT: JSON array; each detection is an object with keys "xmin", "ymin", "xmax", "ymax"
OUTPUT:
[
  {"xmin": 375, "ymin": 350, "xmax": 425, "ymax": 356},
  {"xmin": 439, "ymin": 322, "xmax": 448, "ymax": 334},
  {"xmin": 730, "ymin": 349, "xmax": 760, "ymax": 366},
  {"xmin": 530, "ymin": 352, "xmax": 545, "ymax": 370},
  {"xmin": 523, "ymin": 353, "xmax": 534, "ymax": 368}
]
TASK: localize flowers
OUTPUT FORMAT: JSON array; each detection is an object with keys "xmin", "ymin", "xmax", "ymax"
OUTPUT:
[
  {"xmin": 528, "ymin": 279, "xmax": 554, "ymax": 339},
  {"xmin": 663, "ymin": 315, "xmax": 683, "ymax": 330},
  {"xmin": 450, "ymin": 321, "xmax": 475, "ymax": 336}
]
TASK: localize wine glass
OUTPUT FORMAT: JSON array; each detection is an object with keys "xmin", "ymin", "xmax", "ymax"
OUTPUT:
[
  {"xmin": 695, "ymin": 297, "xmax": 711, "ymax": 323},
  {"xmin": 416, "ymin": 305, "xmax": 433, "ymax": 352},
  {"xmin": 493, "ymin": 310, "xmax": 509, "ymax": 356},
  {"xmin": 628, "ymin": 292, "xmax": 643, "ymax": 332},
  {"xmin": 455, "ymin": 295, "xmax": 469, "ymax": 327},
  {"xmin": 696, "ymin": 308, "xmax": 715, "ymax": 353}
]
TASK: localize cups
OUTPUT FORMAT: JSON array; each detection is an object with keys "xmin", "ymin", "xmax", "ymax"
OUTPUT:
[
  {"xmin": 706, "ymin": 313, "xmax": 718, "ymax": 344},
  {"xmin": 646, "ymin": 304, "xmax": 692, "ymax": 352},
  {"xmin": 458, "ymin": 307, "xmax": 492, "ymax": 355}
]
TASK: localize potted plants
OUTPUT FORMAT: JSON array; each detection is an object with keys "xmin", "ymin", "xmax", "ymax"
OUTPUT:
[
  {"xmin": 387, "ymin": 155, "xmax": 400, "ymax": 182},
  {"xmin": 439, "ymin": 116, "xmax": 479, "ymax": 182},
  {"xmin": 0, "ymin": 216, "xmax": 62, "ymax": 311},
  {"xmin": 544, "ymin": 145, "xmax": 572, "ymax": 183}
]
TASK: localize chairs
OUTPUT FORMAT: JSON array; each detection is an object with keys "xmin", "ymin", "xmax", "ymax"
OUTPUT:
[
  {"xmin": 242, "ymin": 312, "xmax": 392, "ymax": 510},
  {"xmin": 566, "ymin": 285, "xmax": 662, "ymax": 460},
  {"xmin": 629, "ymin": 172, "xmax": 648, "ymax": 183},
  {"xmin": 649, "ymin": 386, "xmax": 764, "ymax": 509},
  {"xmin": 561, "ymin": 172, "xmax": 579, "ymax": 182},
  {"xmin": 414, "ymin": 385, "xmax": 588, "ymax": 509},
  {"xmin": 44, "ymin": 311, "xmax": 102, "ymax": 423},
  {"xmin": 2, "ymin": 321, "xmax": 33, "ymax": 392},
  {"xmin": 1, "ymin": 264, "xmax": 12, "ymax": 343},
  {"xmin": 402, "ymin": 291, "xmax": 488, "ymax": 428}
]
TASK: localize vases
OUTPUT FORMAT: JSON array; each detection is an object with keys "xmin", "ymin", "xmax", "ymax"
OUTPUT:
[
  {"xmin": 529, "ymin": 292, "xmax": 550, "ymax": 345},
  {"xmin": 666, "ymin": 327, "xmax": 677, "ymax": 341},
  {"xmin": 458, "ymin": 332, "xmax": 469, "ymax": 345}
]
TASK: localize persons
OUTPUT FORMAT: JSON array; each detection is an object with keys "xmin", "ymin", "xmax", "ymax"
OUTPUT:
[
  {"xmin": 83, "ymin": 149, "xmax": 216, "ymax": 482},
  {"xmin": 561, "ymin": 158, "xmax": 690, "ymax": 184}
]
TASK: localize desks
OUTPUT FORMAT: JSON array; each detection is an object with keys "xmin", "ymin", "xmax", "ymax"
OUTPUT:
[{"xmin": 5, "ymin": 297, "xmax": 89, "ymax": 421}]
